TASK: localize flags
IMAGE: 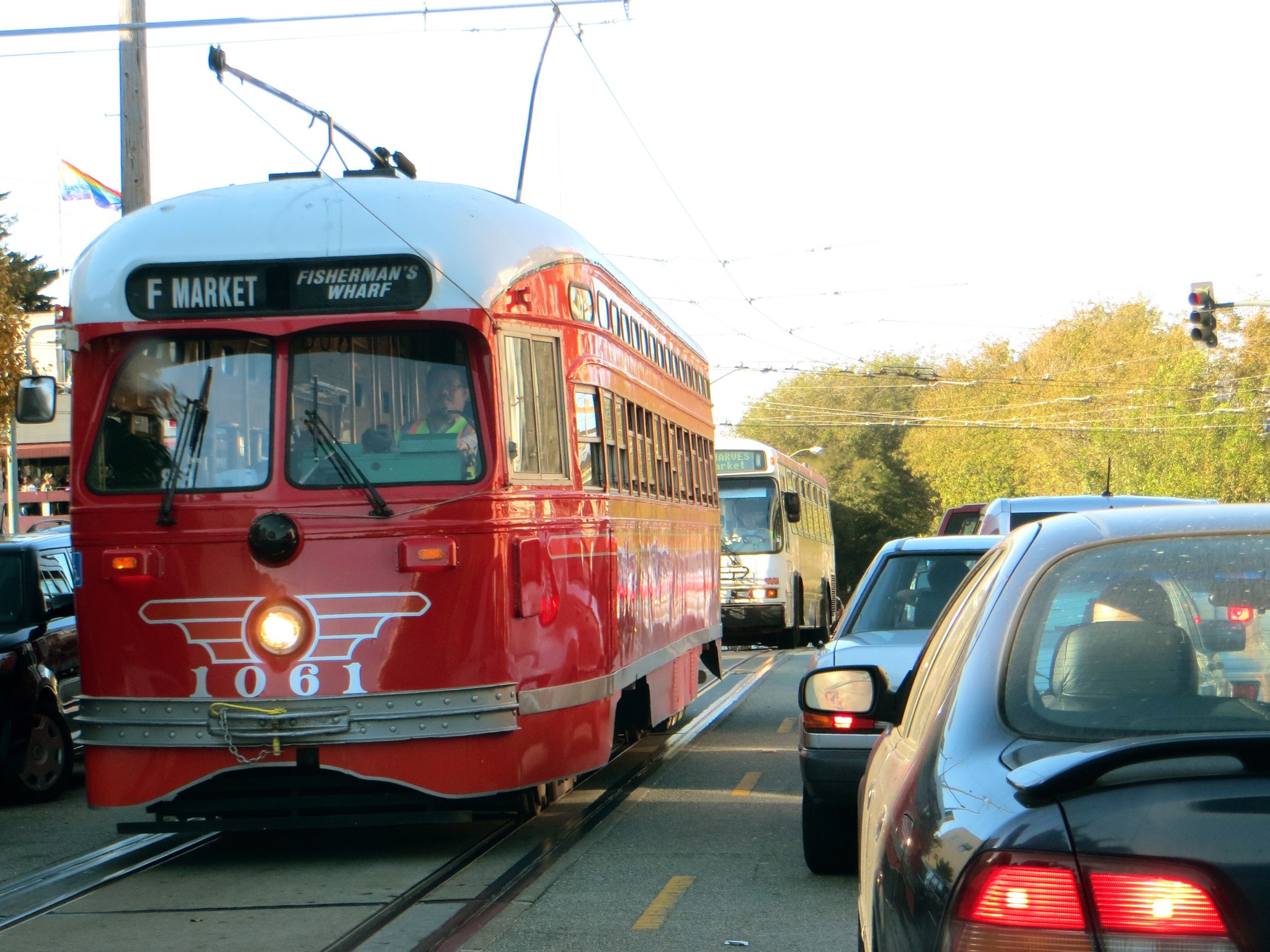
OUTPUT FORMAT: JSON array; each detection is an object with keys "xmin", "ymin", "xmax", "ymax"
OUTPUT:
[{"xmin": 59, "ymin": 158, "xmax": 122, "ymax": 212}]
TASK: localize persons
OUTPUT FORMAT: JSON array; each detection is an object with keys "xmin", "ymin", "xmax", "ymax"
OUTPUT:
[
  {"xmin": 896, "ymin": 556, "xmax": 970, "ymax": 608},
  {"xmin": 377, "ymin": 360, "xmax": 477, "ymax": 465},
  {"xmin": 1, "ymin": 468, "xmax": 70, "ymax": 516},
  {"xmin": 1092, "ymin": 578, "xmax": 1178, "ymax": 626},
  {"xmin": 732, "ymin": 509, "xmax": 780, "ymax": 544}
]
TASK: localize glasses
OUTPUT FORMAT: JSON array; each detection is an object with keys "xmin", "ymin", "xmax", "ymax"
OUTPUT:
[{"xmin": 431, "ymin": 383, "xmax": 461, "ymax": 390}]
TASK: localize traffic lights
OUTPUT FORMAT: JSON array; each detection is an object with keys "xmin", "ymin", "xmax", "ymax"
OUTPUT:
[{"xmin": 1188, "ymin": 289, "xmax": 1218, "ymax": 350}]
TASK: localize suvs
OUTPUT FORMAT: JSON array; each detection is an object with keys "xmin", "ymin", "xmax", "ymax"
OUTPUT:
[{"xmin": 0, "ymin": 518, "xmax": 84, "ymax": 801}]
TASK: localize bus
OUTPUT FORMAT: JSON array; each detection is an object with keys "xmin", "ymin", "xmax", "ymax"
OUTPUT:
[
  {"xmin": 12, "ymin": 4, "xmax": 722, "ymax": 847},
  {"xmin": 713, "ymin": 437, "xmax": 838, "ymax": 647}
]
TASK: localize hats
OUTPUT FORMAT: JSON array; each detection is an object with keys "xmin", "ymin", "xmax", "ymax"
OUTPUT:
[{"xmin": 43, "ymin": 473, "xmax": 53, "ymax": 480}]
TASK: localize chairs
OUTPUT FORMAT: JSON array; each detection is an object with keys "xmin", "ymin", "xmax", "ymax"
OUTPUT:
[
  {"xmin": 913, "ymin": 558, "xmax": 971, "ymax": 629},
  {"xmin": 1094, "ymin": 579, "xmax": 1176, "ymax": 623}
]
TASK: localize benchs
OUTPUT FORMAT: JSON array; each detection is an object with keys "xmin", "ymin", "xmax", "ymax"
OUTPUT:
[{"xmin": 1041, "ymin": 621, "xmax": 1270, "ymax": 718}]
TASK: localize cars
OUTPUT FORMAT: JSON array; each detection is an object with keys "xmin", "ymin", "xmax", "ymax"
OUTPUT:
[{"xmin": 797, "ymin": 491, "xmax": 1267, "ymax": 952}]
